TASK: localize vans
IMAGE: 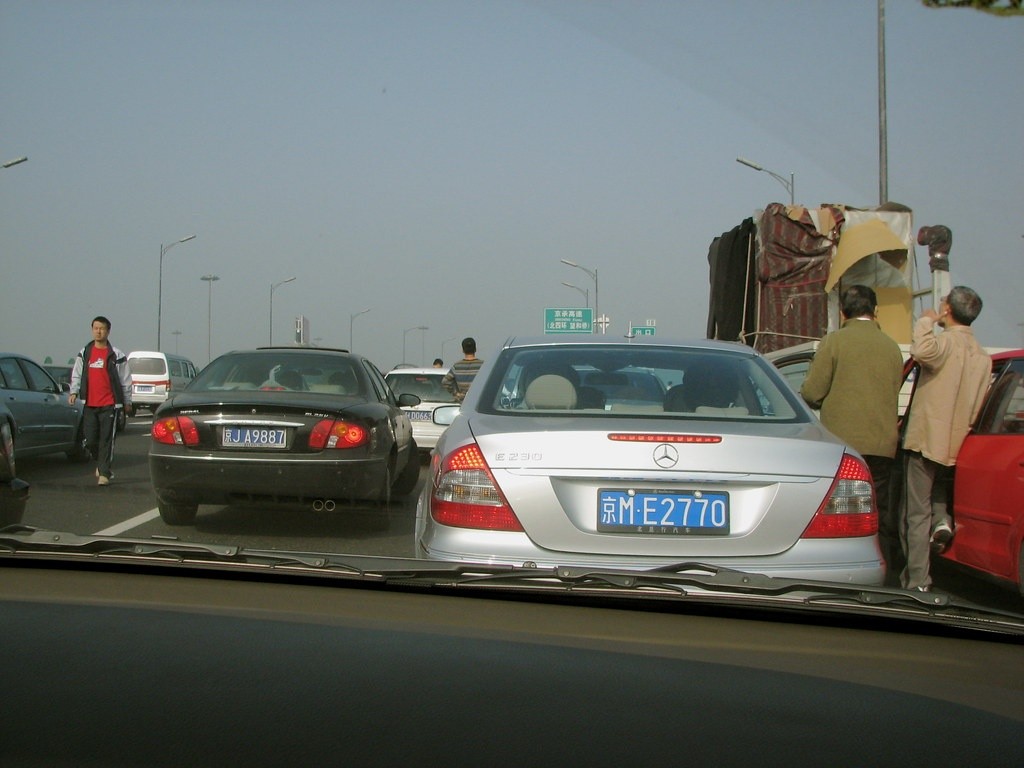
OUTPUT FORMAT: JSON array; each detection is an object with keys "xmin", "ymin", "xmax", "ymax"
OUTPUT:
[{"xmin": 126, "ymin": 350, "xmax": 199, "ymax": 414}]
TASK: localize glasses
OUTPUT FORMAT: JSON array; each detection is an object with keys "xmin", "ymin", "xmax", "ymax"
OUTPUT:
[{"xmin": 940, "ymin": 296, "xmax": 947, "ymax": 302}]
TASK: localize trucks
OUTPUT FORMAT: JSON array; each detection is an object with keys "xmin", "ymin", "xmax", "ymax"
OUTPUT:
[{"xmin": 752, "ymin": 339, "xmax": 1024, "ymax": 423}]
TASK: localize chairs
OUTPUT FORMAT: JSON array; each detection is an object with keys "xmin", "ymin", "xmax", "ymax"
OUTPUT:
[
  {"xmin": 524, "ymin": 373, "xmax": 579, "ymax": 411},
  {"xmin": 311, "ymin": 383, "xmax": 346, "ymax": 394},
  {"xmin": 221, "ymin": 381, "xmax": 255, "ymax": 390}
]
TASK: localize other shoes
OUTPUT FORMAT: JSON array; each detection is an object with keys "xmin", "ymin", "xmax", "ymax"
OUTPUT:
[
  {"xmin": 97, "ymin": 476, "xmax": 110, "ymax": 485},
  {"xmin": 96, "ymin": 466, "xmax": 100, "ymax": 476}
]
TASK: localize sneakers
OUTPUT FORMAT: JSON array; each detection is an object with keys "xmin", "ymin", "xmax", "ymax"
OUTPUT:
[{"xmin": 929, "ymin": 518, "xmax": 953, "ymax": 554}]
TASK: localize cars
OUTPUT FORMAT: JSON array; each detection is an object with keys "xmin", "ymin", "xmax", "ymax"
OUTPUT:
[
  {"xmin": 414, "ymin": 340, "xmax": 889, "ymax": 593},
  {"xmin": 0, "ymin": 351, "xmax": 87, "ymax": 466},
  {"xmin": 148, "ymin": 344, "xmax": 424, "ymax": 525},
  {"xmin": 381, "ymin": 365, "xmax": 465, "ymax": 452},
  {"xmin": 893, "ymin": 347, "xmax": 1024, "ymax": 595},
  {"xmin": 503, "ymin": 364, "xmax": 671, "ymax": 404}
]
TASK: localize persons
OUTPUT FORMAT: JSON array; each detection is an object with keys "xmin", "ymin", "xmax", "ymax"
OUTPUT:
[
  {"xmin": 894, "ymin": 285, "xmax": 993, "ymax": 590},
  {"xmin": 800, "ymin": 284, "xmax": 905, "ymax": 596},
  {"xmin": 432, "ymin": 358, "xmax": 443, "ymax": 368},
  {"xmin": 68, "ymin": 316, "xmax": 134, "ymax": 486},
  {"xmin": 440, "ymin": 338, "xmax": 484, "ymax": 404}
]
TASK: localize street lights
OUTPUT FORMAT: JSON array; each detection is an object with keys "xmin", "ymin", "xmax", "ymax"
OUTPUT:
[
  {"xmin": 561, "ymin": 259, "xmax": 599, "ymax": 337},
  {"xmin": 201, "ymin": 275, "xmax": 220, "ymax": 361},
  {"xmin": 737, "ymin": 157, "xmax": 793, "ymax": 199},
  {"xmin": 171, "ymin": 330, "xmax": 182, "ymax": 350},
  {"xmin": 157, "ymin": 235, "xmax": 197, "ymax": 350},
  {"xmin": 270, "ymin": 277, "xmax": 296, "ymax": 345},
  {"xmin": 442, "ymin": 339, "xmax": 456, "ymax": 361},
  {"xmin": 350, "ymin": 309, "xmax": 370, "ymax": 353},
  {"xmin": 403, "ymin": 326, "xmax": 430, "ymax": 368}
]
